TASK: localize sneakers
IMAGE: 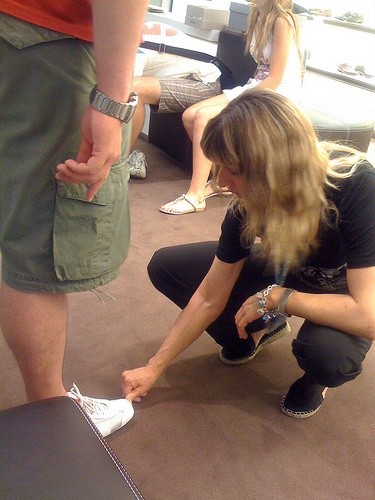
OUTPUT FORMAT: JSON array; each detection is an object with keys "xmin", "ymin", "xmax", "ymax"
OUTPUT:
[
  {"xmin": 66, "ymin": 382, "xmax": 135, "ymax": 438},
  {"xmin": 281, "ymin": 373, "xmax": 328, "ymax": 419},
  {"xmin": 130, "ymin": 150, "xmax": 148, "ymax": 178},
  {"xmin": 220, "ymin": 319, "xmax": 289, "ymax": 365}
]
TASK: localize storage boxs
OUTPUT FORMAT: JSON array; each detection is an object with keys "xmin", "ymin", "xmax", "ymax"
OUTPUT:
[
  {"xmin": 229, "ymin": 1, "xmax": 251, "ymax": 32},
  {"xmin": 185, "ymin": 5, "xmax": 230, "ymax": 29}
]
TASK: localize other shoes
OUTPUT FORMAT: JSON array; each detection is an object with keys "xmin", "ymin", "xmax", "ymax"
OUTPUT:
[
  {"xmin": 335, "ymin": 11, "xmax": 352, "ymax": 21},
  {"xmin": 346, "ymin": 13, "xmax": 364, "ymax": 23},
  {"xmin": 309, "ymin": 8, "xmax": 331, "ymax": 17},
  {"xmin": 355, "ymin": 65, "xmax": 373, "ymax": 78},
  {"xmin": 337, "ymin": 63, "xmax": 359, "ymax": 76}
]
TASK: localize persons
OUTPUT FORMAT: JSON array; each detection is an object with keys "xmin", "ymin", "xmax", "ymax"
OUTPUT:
[
  {"xmin": 0, "ymin": 0, "xmax": 150, "ymax": 439},
  {"xmin": 121, "ymin": 87, "xmax": 375, "ymax": 419},
  {"xmin": 158, "ymin": 0, "xmax": 305, "ymax": 215},
  {"xmin": 128, "ymin": 21, "xmax": 222, "ymax": 178}
]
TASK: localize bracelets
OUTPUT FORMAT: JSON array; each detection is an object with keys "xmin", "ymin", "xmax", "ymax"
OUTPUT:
[{"xmin": 256, "ymin": 284, "xmax": 279, "ymax": 320}]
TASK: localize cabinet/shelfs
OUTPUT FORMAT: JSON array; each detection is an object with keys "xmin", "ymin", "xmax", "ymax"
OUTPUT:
[{"xmin": 306, "ymin": 8, "xmax": 375, "ymax": 92}]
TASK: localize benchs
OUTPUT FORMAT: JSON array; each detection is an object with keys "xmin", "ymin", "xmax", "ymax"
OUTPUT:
[{"xmin": 149, "ymin": 98, "xmax": 375, "ymax": 176}]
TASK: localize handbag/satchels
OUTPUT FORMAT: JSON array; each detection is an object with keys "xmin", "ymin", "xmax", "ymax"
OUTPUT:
[{"xmin": 211, "ymin": 57, "xmax": 236, "ymax": 88}]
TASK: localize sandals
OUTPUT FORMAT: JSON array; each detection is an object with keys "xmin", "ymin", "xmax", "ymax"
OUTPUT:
[
  {"xmin": 205, "ymin": 179, "xmax": 234, "ymax": 200},
  {"xmin": 159, "ymin": 194, "xmax": 206, "ymax": 214}
]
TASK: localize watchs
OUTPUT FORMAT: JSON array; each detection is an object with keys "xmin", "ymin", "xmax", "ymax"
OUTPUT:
[
  {"xmin": 277, "ymin": 288, "xmax": 298, "ymax": 318},
  {"xmin": 89, "ymin": 84, "xmax": 138, "ymax": 124}
]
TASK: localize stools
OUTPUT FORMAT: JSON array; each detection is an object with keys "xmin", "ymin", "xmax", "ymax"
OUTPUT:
[{"xmin": 0, "ymin": 396, "xmax": 145, "ymax": 500}]
